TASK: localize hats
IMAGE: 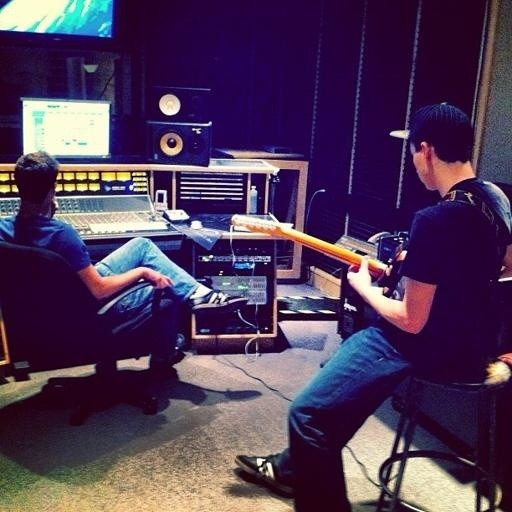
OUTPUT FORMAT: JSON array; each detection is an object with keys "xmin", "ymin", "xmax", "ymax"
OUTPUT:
[{"xmin": 390, "ymin": 102, "xmax": 477, "ymax": 147}]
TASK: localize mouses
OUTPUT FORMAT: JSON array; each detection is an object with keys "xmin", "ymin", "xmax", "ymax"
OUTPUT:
[{"xmin": 191, "ymin": 221, "xmax": 202, "ymax": 230}]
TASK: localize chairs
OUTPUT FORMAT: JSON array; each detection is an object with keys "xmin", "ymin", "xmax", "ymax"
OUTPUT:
[{"xmin": 0, "ymin": 239, "xmax": 160, "ymax": 427}]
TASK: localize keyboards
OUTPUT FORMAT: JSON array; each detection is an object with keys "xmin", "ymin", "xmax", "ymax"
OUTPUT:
[{"xmin": 88, "ymin": 222, "xmax": 167, "ymax": 234}]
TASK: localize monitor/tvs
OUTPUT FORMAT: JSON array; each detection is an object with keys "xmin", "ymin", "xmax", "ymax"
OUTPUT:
[
  {"xmin": 0, "ymin": 0, "xmax": 127, "ymax": 49},
  {"xmin": 19, "ymin": 97, "xmax": 111, "ymax": 160}
]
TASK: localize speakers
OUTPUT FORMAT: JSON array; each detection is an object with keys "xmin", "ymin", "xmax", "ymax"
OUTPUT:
[
  {"xmin": 145, "ymin": 121, "xmax": 212, "ymax": 167},
  {"xmin": 149, "ymin": 84, "xmax": 211, "ymax": 123}
]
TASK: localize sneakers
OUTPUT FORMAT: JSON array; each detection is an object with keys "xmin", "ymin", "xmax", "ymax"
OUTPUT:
[
  {"xmin": 189, "ymin": 290, "xmax": 248, "ymax": 313},
  {"xmin": 150, "ymin": 334, "xmax": 186, "ymax": 371},
  {"xmin": 234, "ymin": 456, "xmax": 296, "ymax": 497}
]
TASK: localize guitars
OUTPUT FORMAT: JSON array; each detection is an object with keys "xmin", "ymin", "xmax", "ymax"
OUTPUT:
[{"xmin": 231, "ymin": 213, "xmax": 411, "ymax": 297}]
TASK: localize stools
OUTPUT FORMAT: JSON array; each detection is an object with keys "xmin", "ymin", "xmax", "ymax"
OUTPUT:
[{"xmin": 376, "ymin": 358, "xmax": 511, "ymax": 512}]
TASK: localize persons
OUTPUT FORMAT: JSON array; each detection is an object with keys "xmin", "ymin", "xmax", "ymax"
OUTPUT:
[
  {"xmin": 234, "ymin": 101, "xmax": 512, "ymax": 512},
  {"xmin": 0, "ymin": 149, "xmax": 248, "ymax": 399}
]
